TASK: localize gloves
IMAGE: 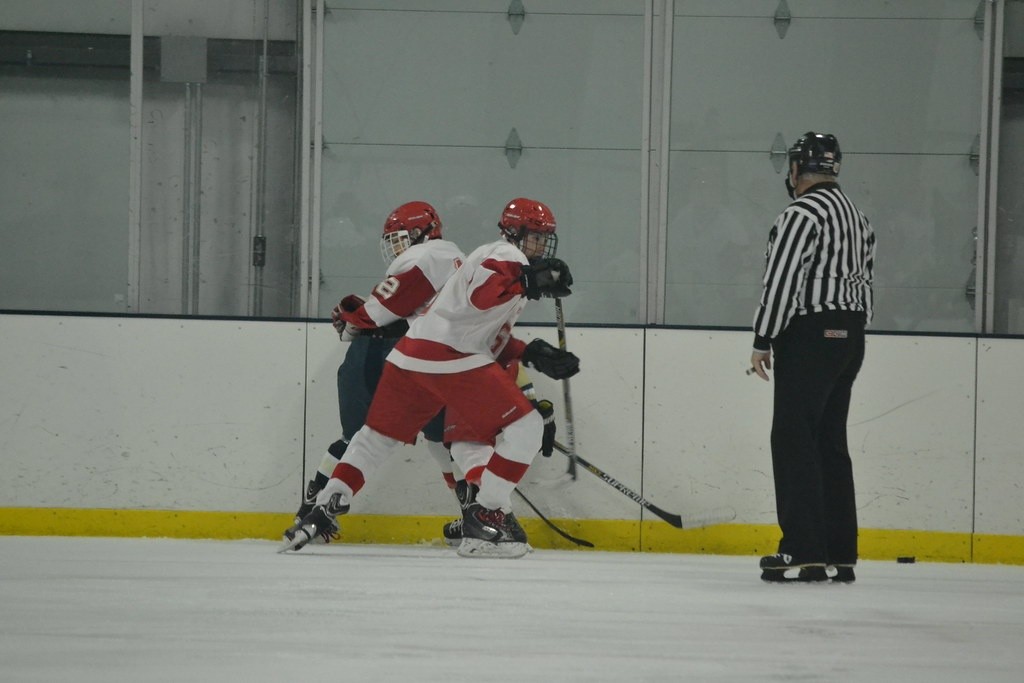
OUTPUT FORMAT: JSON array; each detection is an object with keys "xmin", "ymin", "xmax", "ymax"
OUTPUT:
[
  {"xmin": 520, "ymin": 258, "xmax": 573, "ymax": 300},
  {"xmin": 535, "ymin": 400, "xmax": 557, "ymax": 457},
  {"xmin": 520, "ymin": 383, "xmax": 538, "ymax": 408},
  {"xmin": 521, "ymin": 338, "xmax": 580, "ymax": 380},
  {"xmin": 339, "ymin": 321, "xmax": 361, "ymax": 342}
]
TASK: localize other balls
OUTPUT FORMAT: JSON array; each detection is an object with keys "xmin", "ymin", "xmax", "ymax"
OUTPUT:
[{"xmin": 896, "ymin": 556, "xmax": 916, "ymax": 563}]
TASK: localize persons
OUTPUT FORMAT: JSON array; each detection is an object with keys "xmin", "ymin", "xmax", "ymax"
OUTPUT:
[
  {"xmin": 750, "ymin": 132, "xmax": 877, "ymax": 587},
  {"xmin": 283, "ymin": 314, "xmax": 480, "ymax": 546},
  {"xmin": 330, "ymin": 201, "xmax": 552, "ymax": 505},
  {"xmin": 277, "ymin": 199, "xmax": 580, "ymax": 559}
]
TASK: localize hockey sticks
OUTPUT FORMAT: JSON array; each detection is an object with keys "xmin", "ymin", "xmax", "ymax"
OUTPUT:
[{"xmin": 514, "ymin": 296, "xmax": 738, "ymax": 551}]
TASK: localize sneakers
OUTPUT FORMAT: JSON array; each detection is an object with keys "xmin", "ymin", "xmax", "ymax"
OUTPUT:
[
  {"xmin": 277, "ymin": 480, "xmax": 351, "ymax": 553},
  {"xmin": 443, "ymin": 480, "xmax": 535, "ymax": 554},
  {"xmin": 456, "ymin": 483, "xmax": 527, "ymax": 559},
  {"xmin": 760, "ymin": 552, "xmax": 857, "ymax": 582}
]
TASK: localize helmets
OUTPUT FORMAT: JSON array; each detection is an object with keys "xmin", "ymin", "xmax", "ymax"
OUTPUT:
[
  {"xmin": 785, "ymin": 131, "xmax": 842, "ymax": 200},
  {"xmin": 497, "ymin": 197, "xmax": 558, "ymax": 264},
  {"xmin": 380, "ymin": 201, "xmax": 443, "ymax": 266}
]
